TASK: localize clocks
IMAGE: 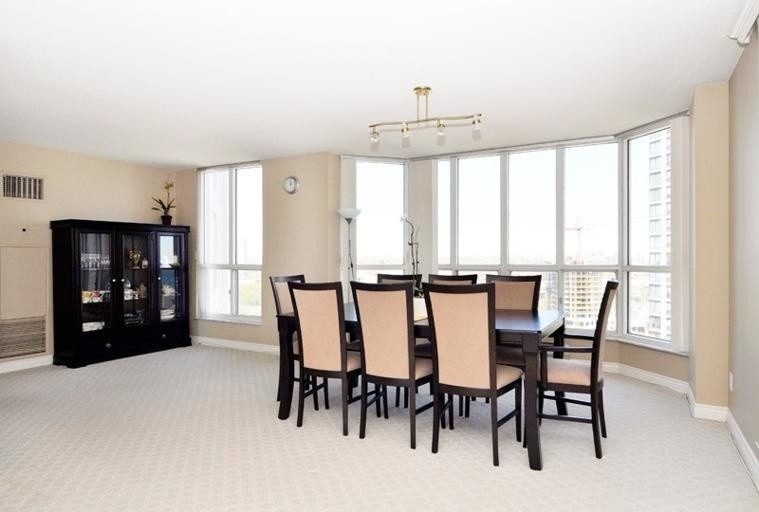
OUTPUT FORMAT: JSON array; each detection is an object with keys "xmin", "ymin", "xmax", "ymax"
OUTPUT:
[{"xmin": 283, "ymin": 176, "xmax": 299, "ymax": 194}]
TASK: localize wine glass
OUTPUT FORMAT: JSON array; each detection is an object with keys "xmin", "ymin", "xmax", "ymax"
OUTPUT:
[{"xmin": 81, "ymin": 254, "xmax": 111, "ymax": 269}]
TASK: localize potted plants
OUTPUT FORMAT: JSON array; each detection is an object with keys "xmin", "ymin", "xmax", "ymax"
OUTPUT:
[{"xmin": 151, "ymin": 181, "xmax": 177, "ymax": 225}]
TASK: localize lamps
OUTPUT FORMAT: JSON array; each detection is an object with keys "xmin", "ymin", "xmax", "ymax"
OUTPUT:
[
  {"xmin": 338, "ymin": 208, "xmax": 362, "ymax": 301},
  {"xmin": 368, "ymin": 85, "xmax": 483, "ymax": 140}
]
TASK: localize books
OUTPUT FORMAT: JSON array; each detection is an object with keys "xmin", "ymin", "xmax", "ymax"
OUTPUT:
[{"xmin": 81, "ymin": 306, "xmax": 176, "ymax": 333}]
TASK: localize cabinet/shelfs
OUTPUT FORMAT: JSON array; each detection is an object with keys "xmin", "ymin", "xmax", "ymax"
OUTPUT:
[{"xmin": 49, "ymin": 218, "xmax": 191, "ymax": 369}]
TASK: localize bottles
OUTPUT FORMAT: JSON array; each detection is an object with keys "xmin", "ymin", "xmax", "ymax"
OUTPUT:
[{"xmin": 142, "ymin": 258, "xmax": 148, "ymax": 269}]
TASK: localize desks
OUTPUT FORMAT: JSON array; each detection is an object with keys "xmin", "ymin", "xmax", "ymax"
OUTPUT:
[{"xmin": 275, "ymin": 303, "xmax": 568, "ymax": 471}]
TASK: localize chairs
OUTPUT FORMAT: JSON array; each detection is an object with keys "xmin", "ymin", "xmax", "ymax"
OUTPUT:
[
  {"xmin": 268, "ymin": 272, "xmax": 333, "ymax": 412},
  {"xmin": 420, "ymin": 281, "xmax": 525, "ymax": 465},
  {"xmin": 350, "ymin": 280, "xmax": 432, "ymax": 449},
  {"xmin": 427, "ymin": 273, "xmax": 478, "ymax": 291},
  {"xmin": 536, "ymin": 279, "xmax": 619, "ymax": 460},
  {"xmin": 485, "ymin": 272, "xmax": 543, "ymax": 312},
  {"xmin": 376, "ymin": 272, "xmax": 422, "ymax": 298},
  {"xmin": 285, "ymin": 278, "xmax": 361, "ymax": 437}
]
